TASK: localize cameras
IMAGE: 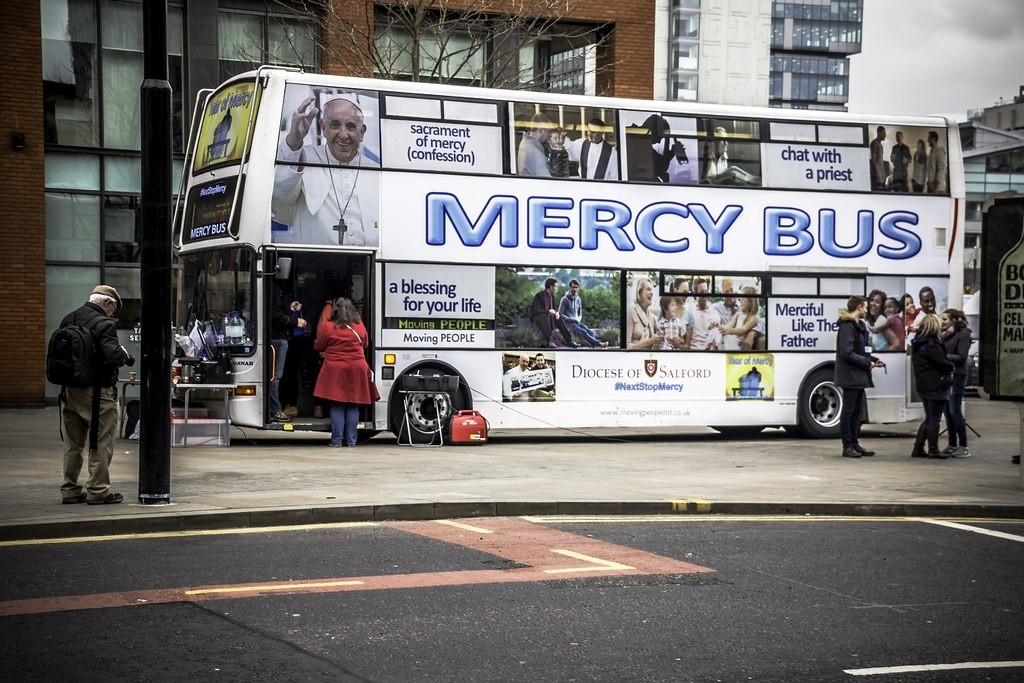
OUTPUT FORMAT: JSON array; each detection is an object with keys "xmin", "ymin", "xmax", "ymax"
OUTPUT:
[{"xmin": 125, "ymin": 354, "xmax": 135, "ymax": 367}]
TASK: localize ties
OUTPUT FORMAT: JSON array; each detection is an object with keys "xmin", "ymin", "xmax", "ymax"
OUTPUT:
[{"xmin": 549, "ymin": 295, "xmax": 553, "ymax": 309}]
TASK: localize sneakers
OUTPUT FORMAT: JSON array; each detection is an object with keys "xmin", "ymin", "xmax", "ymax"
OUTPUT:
[
  {"xmin": 938, "ymin": 445, "xmax": 957, "ymax": 455},
  {"xmin": 270, "ymin": 410, "xmax": 292, "ymax": 423},
  {"xmin": 952, "ymin": 445, "xmax": 970, "ymax": 458}
]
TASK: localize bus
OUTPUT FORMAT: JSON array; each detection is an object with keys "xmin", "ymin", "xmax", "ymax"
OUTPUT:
[{"xmin": 172, "ymin": 63, "xmax": 969, "ymax": 442}]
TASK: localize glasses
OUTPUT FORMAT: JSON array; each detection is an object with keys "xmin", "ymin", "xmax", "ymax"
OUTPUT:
[{"xmin": 110, "ymin": 300, "xmax": 120, "ymax": 314}]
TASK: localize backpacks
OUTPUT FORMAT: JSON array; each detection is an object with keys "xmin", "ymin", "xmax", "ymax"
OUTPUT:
[{"xmin": 46, "ymin": 311, "xmax": 111, "ymax": 385}]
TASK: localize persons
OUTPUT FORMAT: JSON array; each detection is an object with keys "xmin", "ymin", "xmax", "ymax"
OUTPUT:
[
  {"xmin": 833, "ymin": 296, "xmax": 885, "ymax": 458},
  {"xmin": 705, "ymin": 127, "xmax": 728, "ymax": 175},
  {"xmin": 57, "ymin": 285, "xmax": 130, "ymax": 505},
  {"xmin": 627, "ymin": 278, "xmax": 765, "ymax": 350},
  {"xmin": 869, "ymin": 126, "xmax": 886, "ymax": 185},
  {"xmin": 865, "ymin": 286, "xmax": 936, "ymax": 351},
  {"xmin": 270, "ymin": 273, "xmax": 324, "ymax": 421},
  {"xmin": 890, "ymin": 131, "xmax": 912, "ymax": 192},
  {"xmin": 314, "ymin": 297, "xmax": 380, "ymax": 447},
  {"xmin": 517, "ymin": 111, "xmax": 618, "ymax": 180},
  {"xmin": 501, "ymin": 352, "xmax": 555, "ymax": 402},
  {"xmin": 912, "ymin": 139, "xmax": 927, "ymax": 193},
  {"xmin": 910, "ymin": 314, "xmax": 954, "ymax": 459},
  {"xmin": 625, "ymin": 114, "xmax": 685, "ymax": 183},
  {"xmin": 271, "ymin": 93, "xmax": 380, "ymax": 248},
  {"xmin": 531, "ymin": 278, "xmax": 609, "ymax": 348},
  {"xmin": 940, "ymin": 308, "xmax": 972, "ymax": 458},
  {"xmin": 926, "ymin": 131, "xmax": 946, "ymax": 194}
]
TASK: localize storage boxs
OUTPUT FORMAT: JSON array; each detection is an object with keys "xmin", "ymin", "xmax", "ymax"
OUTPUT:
[
  {"xmin": 402, "ymin": 374, "xmax": 425, "ymax": 391},
  {"xmin": 171, "ymin": 416, "xmax": 232, "ymax": 447},
  {"xmin": 424, "ymin": 376, "xmax": 460, "ymax": 393}
]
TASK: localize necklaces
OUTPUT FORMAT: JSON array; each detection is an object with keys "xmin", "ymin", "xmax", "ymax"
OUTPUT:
[{"xmin": 325, "ymin": 143, "xmax": 362, "ymax": 245}]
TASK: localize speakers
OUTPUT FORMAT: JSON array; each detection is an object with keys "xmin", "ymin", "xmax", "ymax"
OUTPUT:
[{"xmin": 401, "ymin": 374, "xmax": 459, "ymax": 392}]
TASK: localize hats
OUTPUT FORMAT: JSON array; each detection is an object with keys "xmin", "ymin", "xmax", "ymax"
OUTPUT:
[
  {"xmin": 323, "ymin": 95, "xmax": 365, "ymax": 117},
  {"xmin": 92, "ymin": 285, "xmax": 123, "ymax": 309}
]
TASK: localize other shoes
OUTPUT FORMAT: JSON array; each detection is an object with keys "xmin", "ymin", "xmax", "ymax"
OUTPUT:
[
  {"xmin": 62, "ymin": 491, "xmax": 88, "ymax": 504},
  {"xmin": 929, "ymin": 447, "xmax": 947, "ymax": 459},
  {"xmin": 88, "ymin": 492, "xmax": 123, "ymax": 505},
  {"xmin": 854, "ymin": 445, "xmax": 875, "ymax": 456},
  {"xmin": 912, "ymin": 447, "xmax": 929, "ymax": 458},
  {"xmin": 842, "ymin": 447, "xmax": 863, "ymax": 458}
]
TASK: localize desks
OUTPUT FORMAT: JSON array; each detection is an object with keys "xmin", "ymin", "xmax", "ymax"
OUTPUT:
[
  {"xmin": 118, "ymin": 378, "xmax": 238, "ymax": 449},
  {"xmin": 397, "ymin": 390, "xmax": 447, "ymax": 447}
]
